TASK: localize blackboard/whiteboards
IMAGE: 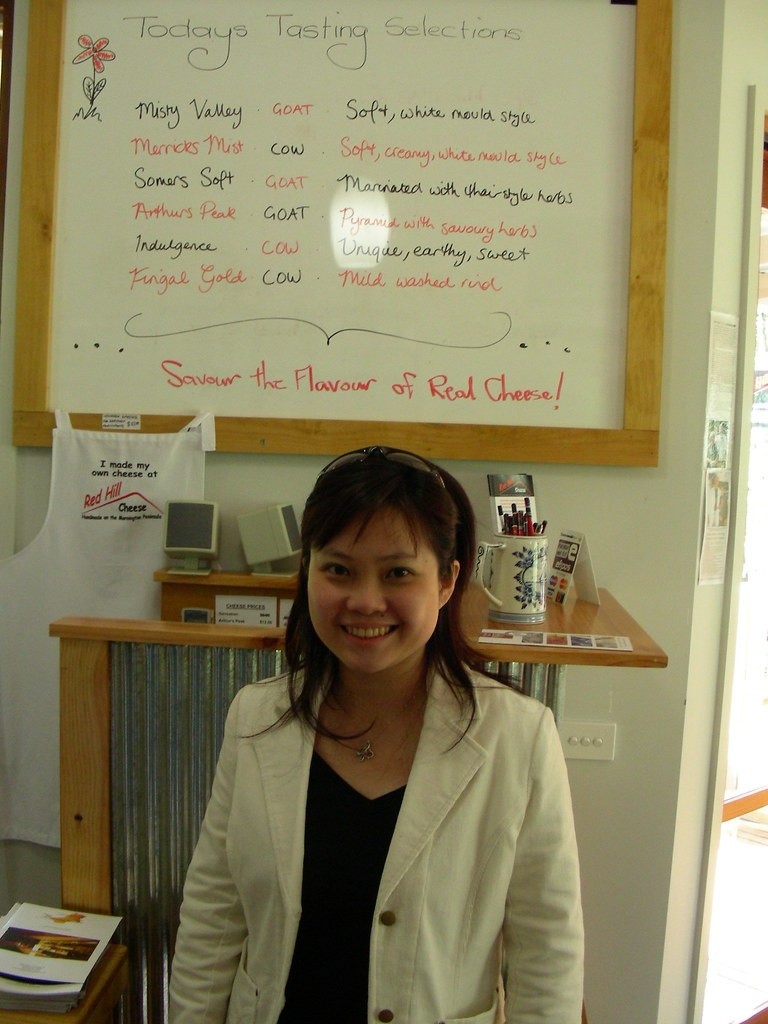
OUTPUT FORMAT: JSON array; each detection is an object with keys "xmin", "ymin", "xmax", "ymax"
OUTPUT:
[{"xmin": 11, "ymin": 0, "xmax": 673, "ymax": 468}]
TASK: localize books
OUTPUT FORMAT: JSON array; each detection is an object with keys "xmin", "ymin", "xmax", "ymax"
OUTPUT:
[{"xmin": 0, "ymin": 900, "xmax": 123, "ymax": 1014}]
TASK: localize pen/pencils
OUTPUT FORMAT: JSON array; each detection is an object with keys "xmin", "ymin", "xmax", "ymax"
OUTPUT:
[{"xmin": 498, "ymin": 494, "xmax": 548, "ymax": 536}]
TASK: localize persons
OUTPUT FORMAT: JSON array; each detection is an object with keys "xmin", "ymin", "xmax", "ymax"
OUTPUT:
[{"xmin": 167, "ymin": 448, "xmax": 586, "ymax": 1024}]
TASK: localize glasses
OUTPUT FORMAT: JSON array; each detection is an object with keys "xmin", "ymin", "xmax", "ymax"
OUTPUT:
[{"xmin": 318, "ymin": 447, "xmax": 447, "ymax": 489}]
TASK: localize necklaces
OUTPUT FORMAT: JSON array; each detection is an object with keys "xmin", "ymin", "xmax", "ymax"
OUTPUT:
[{"xmin": 334, "ymin": 695, "xmax": 415, "ymax": 761}]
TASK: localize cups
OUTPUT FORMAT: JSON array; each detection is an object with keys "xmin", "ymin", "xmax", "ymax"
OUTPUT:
[{"xmin": 469, "ymin": 531, "xmax": 550, "ymax": 624}]
